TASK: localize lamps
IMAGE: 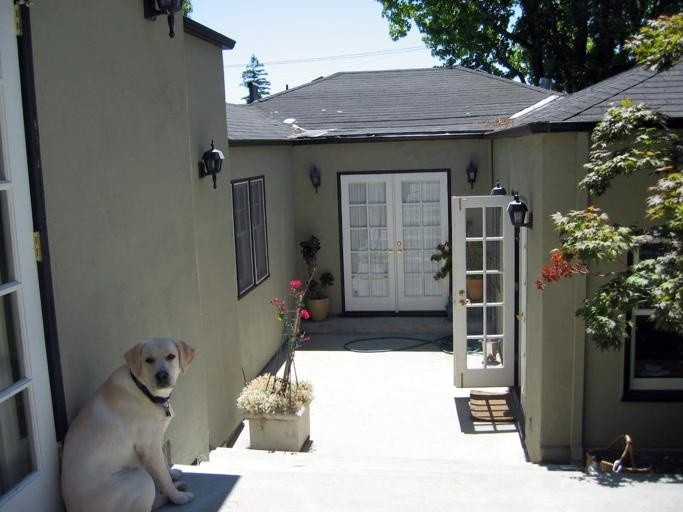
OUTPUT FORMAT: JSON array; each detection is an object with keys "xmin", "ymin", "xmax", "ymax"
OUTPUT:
[
  {"xmin": 507, "ymin": 195, "xmax": 533, "ymax": 229},
  {"xmin": 489, "ymin": 180, "xmax": 507, "ymax": 195},
  {"xmin": 198, "ymin": 139, "xmax": 224, "ymax": 189},
  {"xmin": 308, "ymin": 166, "xmax": 321, "ymax": 194},
  {"xmin": 466, "ymin": 161, "xmax": 478, "ymax": 189}
]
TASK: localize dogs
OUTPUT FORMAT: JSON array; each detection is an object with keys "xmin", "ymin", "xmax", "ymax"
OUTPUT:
[{"xmin": 61, "ymin": 338, "xmax": 196, "ymax": 512}]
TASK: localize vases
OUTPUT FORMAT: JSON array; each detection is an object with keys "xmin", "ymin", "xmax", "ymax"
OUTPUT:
[
  {"xmin": 243, "ymin": 402, "xmax": 309, "ymax": 452},
  {"xmin": 478, "ymin": 339, "xmax": 501, "ymax": 361},
  {"xmin": 430, "ymin": 235, "xmax": 491, "ymax": 299}
]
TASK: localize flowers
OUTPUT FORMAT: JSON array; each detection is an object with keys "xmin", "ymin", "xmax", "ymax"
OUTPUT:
[{"xmin": 270, "ymin": 280, "xmax": 310, "ymax": 402}]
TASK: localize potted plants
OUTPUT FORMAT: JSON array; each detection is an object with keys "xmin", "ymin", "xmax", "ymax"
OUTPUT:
[{"xmin": 298, "ymin": 235, "xmax": 335, "ymax": 321}]
